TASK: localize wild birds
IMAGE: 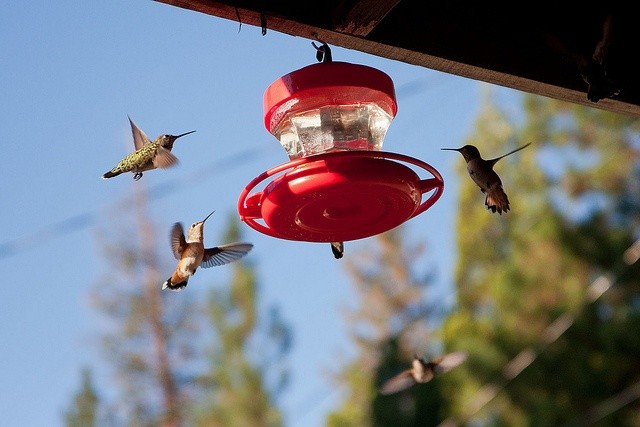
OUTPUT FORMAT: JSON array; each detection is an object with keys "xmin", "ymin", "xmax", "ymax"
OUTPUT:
[
  {"xmin": 100, "ymin": 113, "xmax": 197, "ymax": 182},
  {"xmin": 162, "ymin": 209, "xmax": 254, "ymax": 292},
  {"xmin": 379, "ymin": 348, "xmax": 471, "ymax": 394},
  {"xmin": 441, "ymin": 142, "xmax": 532, "ymax": 217}
]
